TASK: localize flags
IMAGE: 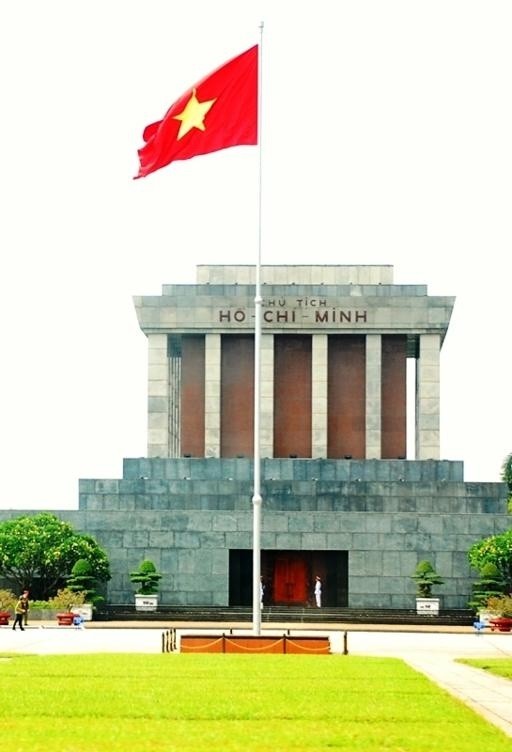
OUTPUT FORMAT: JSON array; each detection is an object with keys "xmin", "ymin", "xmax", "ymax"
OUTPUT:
[{"xmin": 132, "ymin": 43, "xmax": 259, "ymax": 179}]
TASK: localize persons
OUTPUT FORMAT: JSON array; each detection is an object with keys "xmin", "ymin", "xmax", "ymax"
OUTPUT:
[
  {"xmin": 12, "ymin": 595, "xmax": 24, "ymax": 630},
  {"xmin": 314, "ymin": 576, "xmax": 322, "ymax": 608},
  {"xmin": 21, "ymin": 591, "xmax": 30, "ymax": 626},
  {"xmin": 260, "ymin": 576, "xmax": 265, "ymax": 609}
]
